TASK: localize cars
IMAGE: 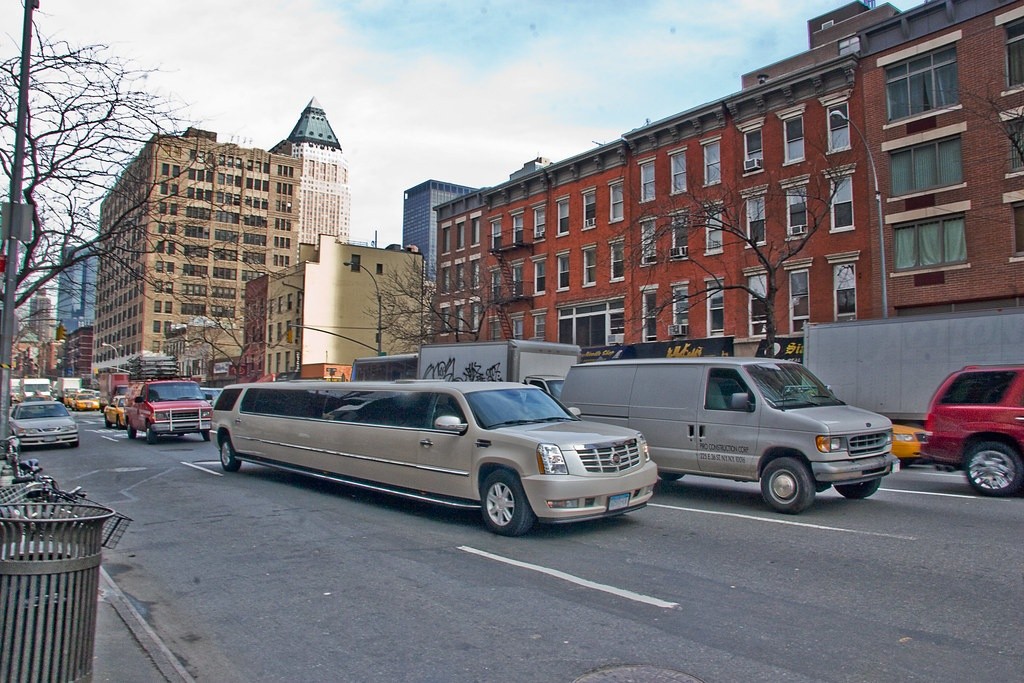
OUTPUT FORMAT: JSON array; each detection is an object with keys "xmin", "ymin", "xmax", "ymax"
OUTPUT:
[
  {"xmin": 9, "ymin": 401, "xmax": 79, "ymax": 453},
  {"xmin": 208, "ymin": 380, "xmax": 658, "ymax": 537},
  {"xmin": 24, "ymin": 391, "xmax": 54, "ymax": 402},
  {"xmin": 200, "ymin": 388, "xmax": 223, "ymax": 408},
  {"xmin": 892, "ymin": 424, "xmax": 926, "ymax": 470},
  {"xmin": 64, "ymin": 390, "xmax": 101, "ymax": 412},
  {"xmin": 104, "ymin": 395, "xmax": 127, "ymax": 430}
]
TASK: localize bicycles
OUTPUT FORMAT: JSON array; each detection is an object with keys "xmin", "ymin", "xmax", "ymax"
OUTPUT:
[{"xmin": 0, "ymin": 434, "xmax": 133, "ymax": 550}]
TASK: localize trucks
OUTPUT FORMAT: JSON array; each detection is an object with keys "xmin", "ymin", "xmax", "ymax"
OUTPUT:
[
  {"xmin": 800, "ymin": 304, "xmax": 1024, "ymax": 422},
  {"xmin": 99, "ymin": 372, "xmax": 129, "ymax": 414},
  {"xmin": 254, "ymin": 363, "xmax": 353, "ymax": 382},
  {"xmin": 416, "ymin": 339, "xmax": 581, "ymax": 402},
  {"xmin": 57, "ymin": 377, "xmax": 81, "ymax": 403}
]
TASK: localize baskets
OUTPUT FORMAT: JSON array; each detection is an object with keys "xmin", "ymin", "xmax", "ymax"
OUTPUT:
[
  {"xmin": 0, "ymin": 482, "xmax": 29, "ymax": 509},
  {"xmin": 73, "ymin": 497, "xmax": 133, "ymax": 550}
]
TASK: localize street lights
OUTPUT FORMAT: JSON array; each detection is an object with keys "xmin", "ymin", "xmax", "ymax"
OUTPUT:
[
  {"xmin": 102, "ymin": 342, "xmax": 118, "ymax": 373},
  {"xmin": 342, "ymin": 261, "xmax": 382, "ymax": 357},
  {"xmin": 829, "ymin": 110, "xmax": 887, "ymax": 318}
]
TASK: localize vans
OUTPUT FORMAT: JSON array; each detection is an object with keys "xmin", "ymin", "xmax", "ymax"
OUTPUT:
[
  {"xmin": 560, "ymin": 357, "xmax": 900, "ymax": 515},
  {"xmin": 22, "ymin": 379, "xmax": 51, "ymax": 401},
  {"xmin": 10, "ymin": 379, "xmax": 22, "ymax": 403}
]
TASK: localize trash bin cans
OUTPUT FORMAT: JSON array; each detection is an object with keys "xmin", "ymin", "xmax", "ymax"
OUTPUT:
[{"xmin": 0, "ymin": 503, "xmax": 116, "ymax": 683}]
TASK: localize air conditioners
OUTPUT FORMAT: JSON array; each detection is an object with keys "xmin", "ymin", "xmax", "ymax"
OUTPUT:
[
  {"xmin": 586, "ymin": 218, "xmax": 596, "ymax": 226},
  {"xmin": 790, "ymin": 225, "xmax": 807, "ymax": 236},
  {"xmin": 744, "ymin": 158, "xmax": 762, "ymax": 172},
  {"xmin": 535, "ymin": 232, "xmax": 543, "ymax": 238},
  {"xmin": 669, "ymin": 246, "xmax": 687, "ymax": 258},
  {"xmin": 668, "ymin": 325, "xmax": 689, "ymax": 335},
  {"xmin": 607, "ymin": 334, "xmax": 624, "ymax": 343}
]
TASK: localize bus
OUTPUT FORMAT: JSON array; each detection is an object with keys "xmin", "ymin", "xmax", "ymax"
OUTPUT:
[{"xmin": 349, "ymin": 352, "xmax": 420, "ymax": 381}]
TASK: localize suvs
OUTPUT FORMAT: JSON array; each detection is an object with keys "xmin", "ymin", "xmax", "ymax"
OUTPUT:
[
  {"xmin": 919, "ymin": 363, "xmax": 1024, "ymax": 498},
  {"xmin": 124, "ymin": 375, "xmax": 213, "ymax": 445}
]
